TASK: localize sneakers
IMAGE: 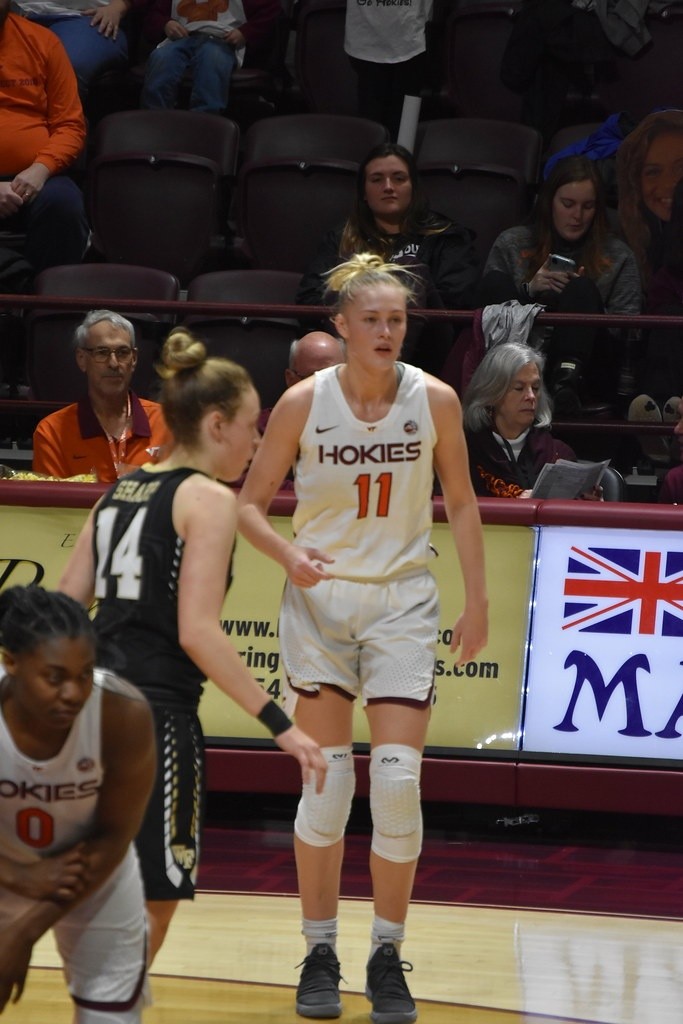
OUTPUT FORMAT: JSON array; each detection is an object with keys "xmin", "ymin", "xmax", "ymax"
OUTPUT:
[
  {"xmin": 295, "ymin": 943, "xmax": 347, "ymax": 1018},
  {"xmin": 366, "ymin": 943, "xmax": 417, "ymax": 1024}
]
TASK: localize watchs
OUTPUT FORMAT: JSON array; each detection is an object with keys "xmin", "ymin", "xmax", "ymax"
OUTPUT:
[{"xmin": 523, "ymin": 282, "xmax": 532, "ymax": 298}]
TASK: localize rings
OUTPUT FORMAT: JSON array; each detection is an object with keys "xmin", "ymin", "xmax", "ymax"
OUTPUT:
[{"xmin": 25, "ymin": 193, "xmax": 30, "ymax": 198}]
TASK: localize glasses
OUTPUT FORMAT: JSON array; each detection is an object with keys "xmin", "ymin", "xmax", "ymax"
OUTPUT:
[{"xmin": 80, "ymin": 347, "xmax": 138, "ymax": 363}]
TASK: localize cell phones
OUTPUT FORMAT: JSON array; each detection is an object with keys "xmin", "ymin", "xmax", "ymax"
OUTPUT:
[{"xmin": 549, "ymin": 254, "xmax": 578, "ymax": 274}]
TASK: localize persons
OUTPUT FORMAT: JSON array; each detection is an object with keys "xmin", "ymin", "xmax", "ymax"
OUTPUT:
[
  {"xmin": 0, "ymin": 0, "xmax": 683, "ymax": 507},
  {"xmin": 58, "ymin": 333, "xmax": 329, "ymax": 972},
  {"xmin": 237, "ymin": 250, "xmax": 491, "ymax": 1024},
  {"xmin": 0, "ymin": 583, "xmax": 156, "ymax": 1024}
]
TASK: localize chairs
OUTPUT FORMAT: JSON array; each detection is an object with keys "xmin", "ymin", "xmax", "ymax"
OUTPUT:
[{"xmin": 19, "ymin": 0, "xmax": 683, "ymax": 503}]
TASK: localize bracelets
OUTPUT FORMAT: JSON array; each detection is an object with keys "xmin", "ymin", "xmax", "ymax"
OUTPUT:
[{"xmin": 257, "ymin": 700, "xmax": 294, "ymax": 735}]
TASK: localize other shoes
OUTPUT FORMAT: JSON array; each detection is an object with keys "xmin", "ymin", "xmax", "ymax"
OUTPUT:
[
  {"xmin": 659, "ymin": 394, "xmax": 683, "ymax": 462},
  {"xmin": 552, "ymin": 358, "xmax": 584, "ymax": 439},
  {"xmin": 628, "ymin": 394, "xmax": 672, "ymax": 464}
]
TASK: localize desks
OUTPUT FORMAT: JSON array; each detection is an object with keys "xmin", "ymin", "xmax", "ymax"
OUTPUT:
[{"xmin": 1, "ymin": 476, "xmax": 681, "ymax": 821}]
